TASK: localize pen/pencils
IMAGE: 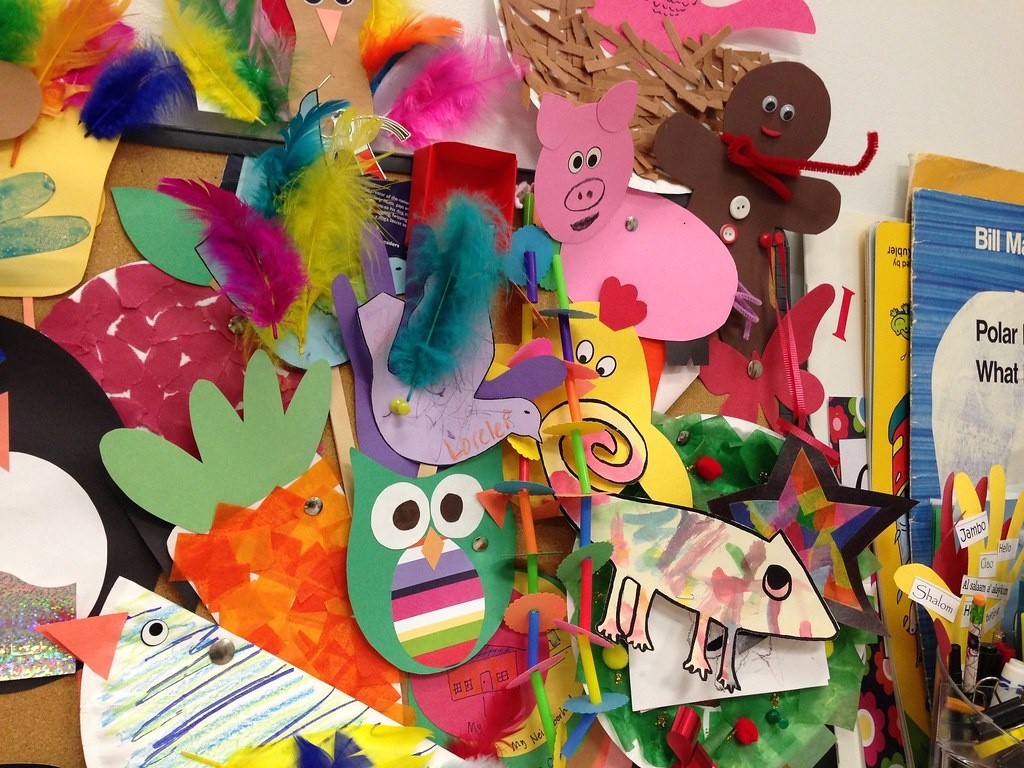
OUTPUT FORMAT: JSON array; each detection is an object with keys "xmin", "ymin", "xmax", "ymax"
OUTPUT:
[
  {"xmin": 1013, "ymin": 575, "xmax": 1024, "ymax": 662},
  {"xmin": 948, "ymin": 591, "xmax": 987, "ymax": 741}
]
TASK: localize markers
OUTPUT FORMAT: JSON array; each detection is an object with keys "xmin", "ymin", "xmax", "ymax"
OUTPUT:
[{"xmin": 974, "ymin": 641, "xmax": 1024, "ymax": 708}]
